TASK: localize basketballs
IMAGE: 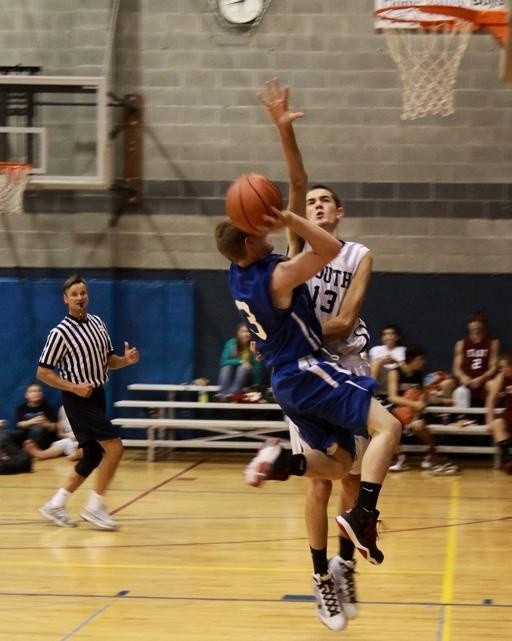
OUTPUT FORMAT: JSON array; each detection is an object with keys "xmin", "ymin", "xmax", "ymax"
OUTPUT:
[{"xmin": 226, "ymin": 173, "xmax": 283, "ymax": 234}]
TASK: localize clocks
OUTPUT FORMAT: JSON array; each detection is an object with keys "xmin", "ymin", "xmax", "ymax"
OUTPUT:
[{"xmin": 209, "ymin": 1, "xmax": 273, "ymax": 29}]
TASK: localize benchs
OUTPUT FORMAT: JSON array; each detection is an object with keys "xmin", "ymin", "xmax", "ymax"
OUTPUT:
[{"xmin": 109, "ymin": 380, "xmax": 509, "ymax": 477}]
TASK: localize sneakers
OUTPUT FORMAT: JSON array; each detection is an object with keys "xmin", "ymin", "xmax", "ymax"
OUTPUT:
[
  {"xmin": 388, "ymin": 454, "xmax": 433, "ymax": 470},
  {"xmin": 39, "ymin": 501, "xmax": 119, "ymax": 530},
  {"xmin": 336, "ymin": 503, "xmax": 383, "ymax": 565},
  {"xmin": 244, "ymin": 438, "xmax": 291, "ymax": 486},
  {"xmin": 313, "ymin": 555, "xmax": 357, "ymax": 632}
]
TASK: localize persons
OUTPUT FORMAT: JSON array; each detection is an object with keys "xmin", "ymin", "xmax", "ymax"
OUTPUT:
[
  {"xmin": 216, "ymin": 206, "xmax": 400, "ymax": 566},
  {"xmin": 36, "ymin": 274, "xmax": 140, "ymax": 531},
  {"xmin": 256, "ymin": 76, "xmax": 376, "ymax": 630},
  {"xmin": 24, "ymin": 397, "xmax": 85, "ymax": 460},
  {"xmin": 14, "ymin": 384, "xmax": 59, "ymax": 458},
  {"xmin": 214, "ymin": 317, "xmax": 511, "ymax": 470}
]
